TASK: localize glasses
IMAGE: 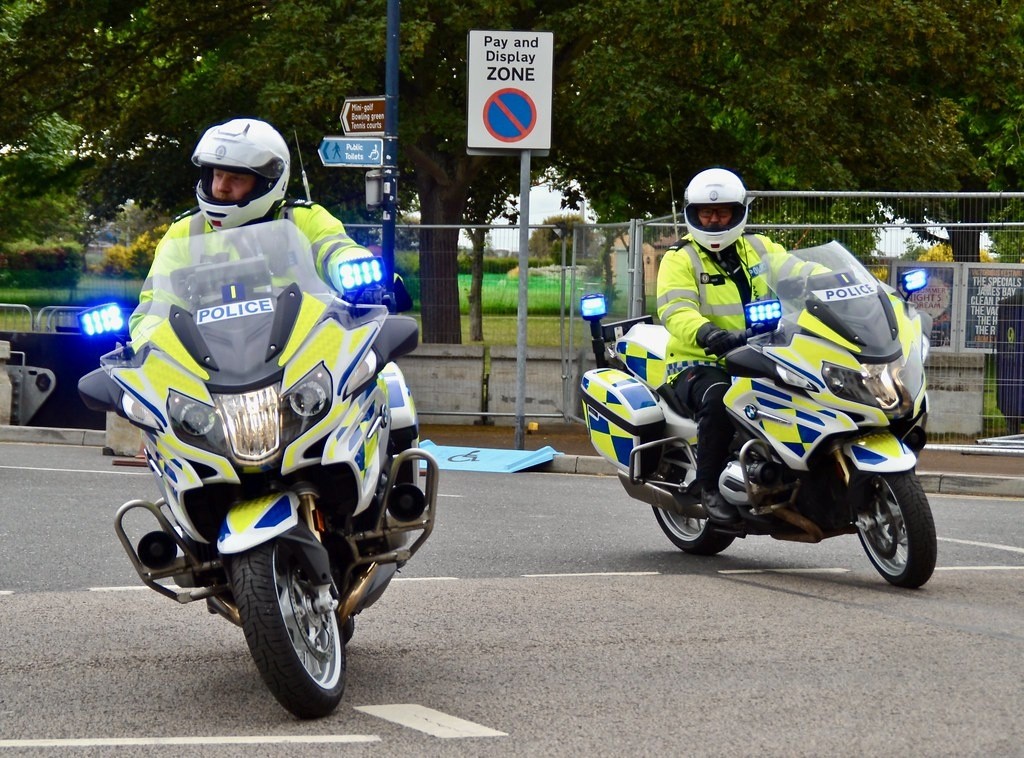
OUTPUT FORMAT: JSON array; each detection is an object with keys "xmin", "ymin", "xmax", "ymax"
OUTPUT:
[{"xmin": 698, "ymin": 206, "xmax": 733, "ymax": 219}]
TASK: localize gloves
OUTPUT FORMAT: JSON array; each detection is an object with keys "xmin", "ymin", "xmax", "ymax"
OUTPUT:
[
  {"xmin": 704, "ymin": 327, "xmax": 738, "ymax": 358},
  {"xmin": 343, "ymin": 286, "xmax": 377, "ymax": 317}
]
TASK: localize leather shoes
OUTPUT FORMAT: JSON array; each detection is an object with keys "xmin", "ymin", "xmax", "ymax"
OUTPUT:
[{"xmin": 701, "ymin": 489, "xmax": 733, "ymax": 522}]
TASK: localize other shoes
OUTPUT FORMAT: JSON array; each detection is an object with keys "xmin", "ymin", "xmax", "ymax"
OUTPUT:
[{"xmin": 385, "ymin": 507, "xmax": 429, "ymax": 527}]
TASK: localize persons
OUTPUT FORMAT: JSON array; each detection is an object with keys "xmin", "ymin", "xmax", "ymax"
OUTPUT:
[
  {"xmin": 127, "ymin": 117, "xmax": 386, "ymax": 614},
  {"xmin": 655, "ymin": 169, "xmax": 841, "ymax": 519},
  {"xmin": 367, "ymin": 243, "xmax": 405, "ymax": 315}
]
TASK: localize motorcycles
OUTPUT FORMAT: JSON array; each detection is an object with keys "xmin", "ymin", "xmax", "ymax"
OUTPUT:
[
  {"xmin": 76, "ymin": 218, "xmax": 440, "ymax": 721},
  {"xmin": 578, "ymin": 237, "xmax": 937, "ymax": 590}
]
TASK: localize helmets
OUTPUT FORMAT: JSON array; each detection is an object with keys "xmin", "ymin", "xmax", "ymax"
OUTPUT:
[
  {"xmin": 681, "ymin": 168, "xmax": 755, "ymax": 253},
  {"xmin": 191, "ymin": 118, "xmax": 290, "ymax": 231}
]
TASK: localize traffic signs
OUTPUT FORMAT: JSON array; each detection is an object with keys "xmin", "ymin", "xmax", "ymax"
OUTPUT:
[
  {"xmin": 318, "ymin": 137, "xmax": 385, "ymax": 169},
  {"xmin": 341, "ymin": 97, "xmax": 384, "ymax": 137}
]
